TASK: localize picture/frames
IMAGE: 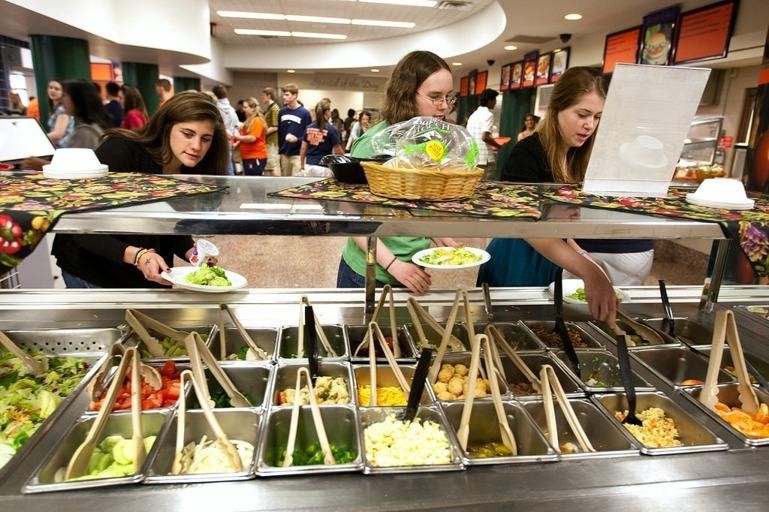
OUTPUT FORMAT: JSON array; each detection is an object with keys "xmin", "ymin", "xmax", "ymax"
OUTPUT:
[
  {"xmin": 500, "ymin": 62, "xmax": 511, "ymax": 92},
  {"xmin": 534, "ymin": 50, "xmax": 551, "ymax": 87},
  {"xmin": 510, "ymin": 59, "xmax": 522, "ymax": 92},
  {"xmin": 476, "ymin": 69, "xmax": 489, "ymax": 94},
  {"xmin": 549, "ymin": 45, "xmax": 571, "ymax": 85},
  {"xmin": 670, "ymin": 0, "xmax": 740, "ymax": 65},
  {"xmin": 520, "ymin": 50, "xmax": 539, "ymax": 90},
  {"xmin": 602, "ymin": 23, "xmax": 641, "ymax": 76},
  {"xmin": 636, "ymin": 3, "xmax": 682, "ymax": 66},
  {"xmin": 459, "ymin": 74, "xmax": 469, "ymax": 97},
  {"xmin": 468, "ymin": 70, "xmax": 475, "ymax": 94}
]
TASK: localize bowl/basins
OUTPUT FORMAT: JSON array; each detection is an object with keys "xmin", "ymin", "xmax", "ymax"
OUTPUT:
[
  {"xmin": 518, "ymin": 397, "xmax": 642, "ymax": 461},
  {"xmin": 438, "ymin": 399, "xmax": 561, "ymax": 467},
  {"xmin": 116, "ymin": 325, "xmax": 218, "ymax": 361},
  {"xmin": 425, "ymin": 354, "xmax": 515, "ymax": 407},
  {"xmin": 20, "ymin": 408, "xmax": 176, "ymax": 495},
  {"xmin": 589, "ymin": 391, "xmax": 730, "ymax": 456},
  {"xmin": 186, "ymin": 361, "xmax": 275, "ymax": 415},
  {"xmin": 641, "ymin": 317, "xmax": 730, "ymax": 348},
  {"xmin": 460, "ymin": 319, "xmax": 547, "ymax": 354},
  {"xmin": 351, "ymin": 362, "xmax": 439, "ymax": 408},
  {"xmin": 207, "ymin": 326, "xmax": 282, "ymax": 363},
  {"xmin": 320, "ymin": 154, "xmax": 391, "ymax": 183},
  {"xmin": 1, "ymin": 327, "xmax": 124, "ymax": 486},
  {"xmin": 680, "ymin": 382, "xmax": 769, "ymax": 446},
  {"xmin": 587, "ymin": 316, "xmax": 683, "ymax": 348},
  {"xmin": 493, "ymin": 352, "xmax": 588, "ymax": 399},
  {"xmin": 627, "ymin": 345, "xmax": 738, "ymax": 390},
  {"xmin": 345, "ymin": 323, "xmax": 418, "ymax": 363},
  {"xmin": 141, "ymin": 407, "xmax": 266, "ymax": 485},
  {"xmin": 405, "ymin": 319, "xmax": 484, "ymax": 358},
  {"xmin": 356, "ymin": 405, "xmax": 468, "ymax": 475},
  {"xmin": 548, "ymin": 349, "xmax": 658, "ymax": 392},
  {"xmin": 523, "ymin": 319, "xmax": 607, "ymax": 351},
  {"xmin": 692, "ymin": 346, "xmax": 769, "ymax": 396},
  {"xmin": 84, "ymin": 363, "xmax": 194, "ymax": 414},
  {"xmin": 277, "ymin": 323, "xmax": 349, "ymax": 361}
]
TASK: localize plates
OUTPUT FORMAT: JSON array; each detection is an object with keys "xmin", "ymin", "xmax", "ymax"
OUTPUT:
[
  {"xmin": 687, "ymin": 179, "xmax": 754, "ymax": 210},
  {"xmin": 43, "ymin": 148, "xmax": 108, "ymax": 179},
  {"xmin": 411, "ymin": 247, "xmax": 491, "ymax": 269},
  {"xmin": 161, "ymin": 266, "xmax": 248, "ymax": 292},
  {"xmin": 490, "ymin": 137, "xmax": 511, "ymax": 151},
  {"xmin": 548, "ymin": 278, "xmax": 628, "ymax": 304}
]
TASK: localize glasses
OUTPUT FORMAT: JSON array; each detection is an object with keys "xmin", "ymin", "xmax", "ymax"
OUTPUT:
[{"xmin": 415, "ymin": 90, "xmax": 457, "ymax": 106}]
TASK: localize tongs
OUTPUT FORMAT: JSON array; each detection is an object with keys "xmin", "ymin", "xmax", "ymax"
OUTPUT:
[
  {"xmin": 91, "ymin": 343, "xmax": 162, "ymax": 402},
  {"xmin": 297, "ymin": 296, "xmax": 338, "ymax": 358},
  {"xmin": 699, "ymin": 308, "xmax": 760, "ymax": 415},
  {"xmin": 283, "ymin": 367, "xmax": 336, "ymax": 466},
  {"xmin": 456, "ymin": 333, "xmax": 518, "ymax": 457},
  {"xmin": 613, "ymin": 308, "xmax": 665, "ymax": 346},
  {"xmin": 125, "ymin": 308, "xmax": 191, "ymax": 357},
  {"xmin": 172, "ymin": 369, "xmax": 243, "ymax": 475},
  {"xmin": 540, "ymin": 364, "xmax": 595, "ymax": 453},
  {"xmin": 484, "ymin": 324, "xmax": 543, "ymax": 394},
  {"xmin": 0, "ymin": 331, "xmax": 44, "ymax": 374},
  {"xmin": 64, "ymin": 346, "xmax": 147, "ymax": 480},
  {"xmin": 429, "ymin": 288, "xmax": 487, "ymax": 381},
  {"xmin": 219, "ymin": 303, "xmax": 267, "ymax": 360},
  {"xmin": 184, "ymin": 331, "xmax": 253, "ymax": 409},
  {"xmin": 368, "ymin": 321, "xmax": 410, "ymax": 406},
  {"xmin": 355, "ymin": 284, "xmax": 402, "ymax": 359},
  {"xmin": 406, "ymin": 296, "xmax": 467, "ymax": 354}
]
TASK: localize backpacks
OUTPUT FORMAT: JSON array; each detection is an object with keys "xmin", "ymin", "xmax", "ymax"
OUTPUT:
[{"xmin": 341, "ymin": 130, "xmax": 347, "ymax": 142}]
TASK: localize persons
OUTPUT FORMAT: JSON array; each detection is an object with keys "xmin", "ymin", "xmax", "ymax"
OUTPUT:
[
  {"xmin": 26, "ymin": 96, "xmax": 40, "ymax": 121},
  {"xmin": 518, "ymin": 113, "xmax": 540, "ymax": 140},
  {"xmin": 278, "ymin": 84, "xmax": 312, "ymax": 177},
  {"xmin": 332, "ymin": 109, "xmax": 346, "ymax": 149},
  {"xmin": 44, "ymin": 81, "xmax": 75, "ymax": 148},
  {"xmin": 337, "ymin": 50, "xmax": 465, "ymax": 295},
  {"xmin": 563, "ymin": 239, "xmax": 655, "ymax": 286},
  {"xmin": 536, "ymin": 56, "xmax": 549, "ymax": 85},
  {"xmin": 501, "ymin": 67, "xmax": 509, "ymax": 89},
  {"xmin": 50, "ymin": 91, "xmax": 230, "ymax": 289},
  {"xmin": 153, "ymin": 80, "xmax": 173, "ymax": 109},
  {"xmin": 119, "ymin": 84, "xmax": 149, "ymax": 130},
  {"xmin": 261, "ymin": 87, "xmax": 281, "ymax": 177},
  {"xmin": 522, "ymin": 63, "xmax": 534, "ymax": 87},
  {"xmin": 57, "ymin": 79, "xmax": 110, "ymax": 152},
  {"xmin": 476, "ymin": 66, "xmax": 618, "ymax": 329},
  {"xmin": 211, "ymin": 85, "xmax": 238, "ymax": 176},
  {"xmin": 104, "ymin": 82, "xmax": 127, "ymax": 128},
  {"xmin": 345, "ymin": 108, "xmax": 355, "ymax": 133},
  {"xmin": 236, "ymin": 99, "xmax": 246, "ymax": 123},
  {"xmin": 296, "ymin": 100, "xmax": 344, "ymax": 178},
  {"xmin": 344, "ymin": 111, "xmax": 371, "ymax": 153},
  {"xmin": 467, "ymin": 89, "xmax": 509, "ymax": 182},
  {"xmin": 234, "ymin": 97, "xmax": 268, "ymax": 176},
  {"xmin": 511, "ymin": 66, "xmax": 521, "ymax": 88}
]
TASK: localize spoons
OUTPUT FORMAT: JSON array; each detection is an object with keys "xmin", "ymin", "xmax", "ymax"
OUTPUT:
[
  {"xmin": 555, "ymin": 314, "xmax": 582, "ymax": 380},
  {"xmin": 615, "ymin": 334, "xmax": 642, "ymax": 427},
  {"xmin": 304, "ymin": 306, "xmax": 318, "ymax": 388},
  {"xmin": 657, "ymin": 279, "xmax": 677, "ymax": 338},
  {"xmin": 401, "ymin": 348, "xmax": 433, "ymax": 423}
]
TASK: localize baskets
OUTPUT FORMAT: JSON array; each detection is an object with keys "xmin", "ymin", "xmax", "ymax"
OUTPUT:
[{"xmin": 360, "ymin": 161, "xmax": 485, "ymax": 201}]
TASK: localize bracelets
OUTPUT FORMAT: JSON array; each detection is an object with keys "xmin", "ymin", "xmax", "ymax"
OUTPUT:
[
  {"xmin": 384, "ymin": 257, "xmax": 396, "ymax": 271},
  {"xmin": 132, "ymin": 247, "xmax": 152, "ymax": 266}
]
